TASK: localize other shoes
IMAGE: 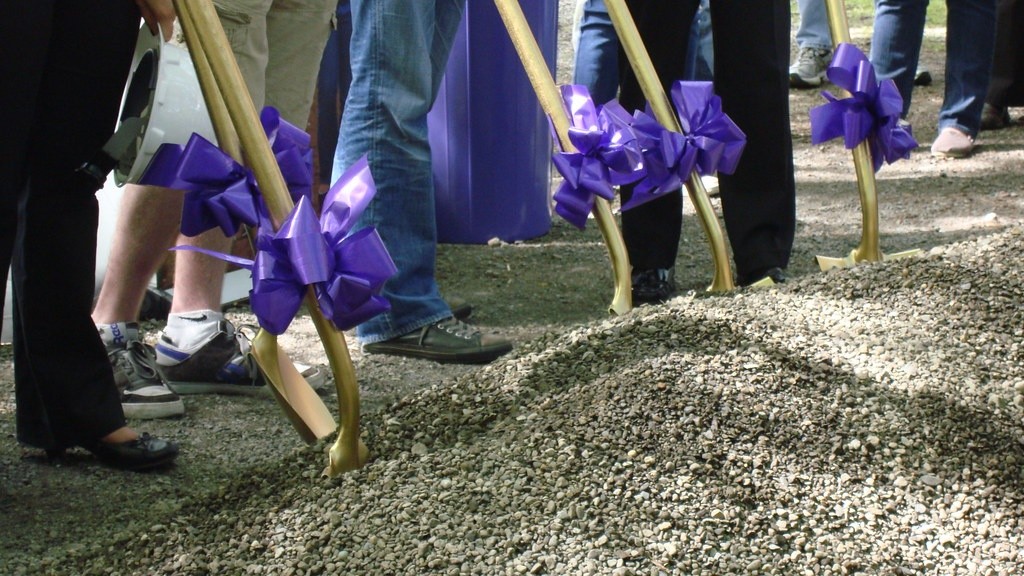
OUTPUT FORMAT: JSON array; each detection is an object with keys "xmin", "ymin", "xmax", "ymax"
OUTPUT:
[{"xmin": 979, "ymin": 103, "xmax": 1010, "ymax": 130}]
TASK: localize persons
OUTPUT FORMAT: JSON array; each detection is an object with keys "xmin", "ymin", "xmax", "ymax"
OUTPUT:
[
  {"xmin": 573, "ymin": 0, "xmax": 715, "ymax": 199},
  {"xmin": 89, "ymin": 0, "xmax": 338, "ymax": 421},
  {"xmin": 789, "ymin": 0, "xmax": 1024, "ymax": 161},
  {"xmin": 617, "ymin": 0, "xmax": 797, "ymax": 307},
  {"xmin": 330, "ymin": 0, "xmax": 513, "ymax": 365},
  {"xmin": 0, "ymin": 0, "xmax": 191, "ymax": 472}
]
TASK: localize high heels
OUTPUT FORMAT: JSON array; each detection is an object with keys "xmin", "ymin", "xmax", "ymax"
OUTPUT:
[{"xmin": 45, "ymin": 432, "xmax": 179, "ymax": 469}]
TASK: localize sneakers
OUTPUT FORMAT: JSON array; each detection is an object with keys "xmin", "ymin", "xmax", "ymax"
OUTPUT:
[
  {"xmin": 154, "ymin": 319, "xmax": 325, "ymax": 400},
  {"xmin": 931, "ymin": 127, "xmax": 974, "ymax": 157},
  {"xmin": 92, "ymin": 321, "xmax": 184, "ymax": 420},
  {"xmin": 788, "ymin": 47, "xmax": 833, "ymax": 89},
  {"xmin": 441, "ymin": 295, "xmax": 471, "ymax": 319},
  {"xmin": 362, "ymin": 314, "xmax": 513, "ymax": 365}
]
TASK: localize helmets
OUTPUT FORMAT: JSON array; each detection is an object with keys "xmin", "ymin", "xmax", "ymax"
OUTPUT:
[{"xmin": 114, "ymin": 20, "xmax": 219, "ymax": 187}]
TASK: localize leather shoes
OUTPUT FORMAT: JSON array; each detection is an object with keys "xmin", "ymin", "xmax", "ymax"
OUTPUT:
[
  {"xmin": 630, "ymin": 266, "xmax": 675, "ymax": 307},
  {"xmin": 737, "ymin": 267, "xmax": 785, "ymax": 286}
]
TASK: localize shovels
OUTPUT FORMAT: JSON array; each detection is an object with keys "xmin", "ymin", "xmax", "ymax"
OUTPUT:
[
  {"xmin": 492, "ymin": 0, "xmax": 636, "ymax": 320},
  {"xmin": 598, "ymin": 0, "xmax": 776, "ymax": 298},
  {"xmin": 814, "ymin": 0, "xmax": 925, "ymax": 274},
  {"xmin": 170, "ymin": 0, "xmax": 380, "ymax": 486}
]
